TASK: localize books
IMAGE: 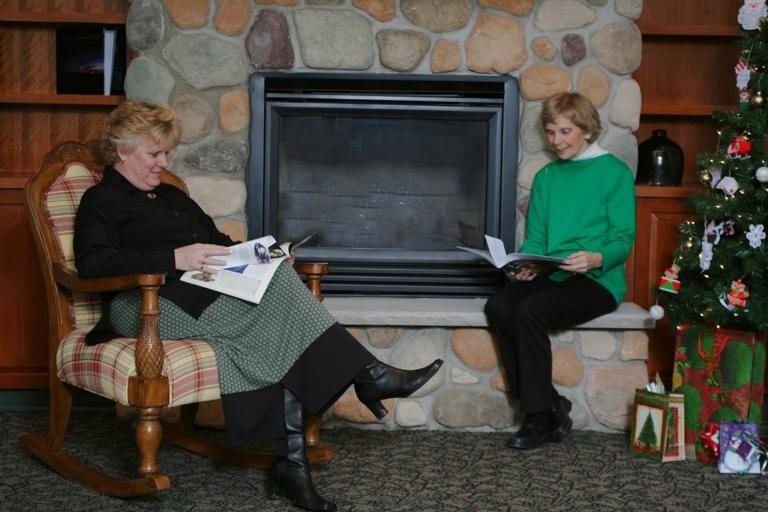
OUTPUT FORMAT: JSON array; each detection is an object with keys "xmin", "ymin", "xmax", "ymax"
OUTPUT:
[
  {"xmin": 179, "ymin": 231, "xmax": 318, "ymax": 305},
  {"xmin": 457, "ymin": 232, "xmax": 567, "ymax": 277}
]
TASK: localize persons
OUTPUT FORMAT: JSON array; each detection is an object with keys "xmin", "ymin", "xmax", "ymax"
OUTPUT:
[
  {"xmin": 483, "ymin": 90, "xmax": 637, "ymax": 450},
  {"xmin": 72, "ymin": 97, "xmax": 443, "ymax": 511}
]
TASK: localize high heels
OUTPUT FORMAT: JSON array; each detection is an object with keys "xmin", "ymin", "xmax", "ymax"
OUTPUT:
[
  {"xmin": 352, "ymin": 357, "xmax": 443, "ymax": 421},
  {"xmin": 266, "ymin": 449, "xmax": 338, "ymax": 512}
]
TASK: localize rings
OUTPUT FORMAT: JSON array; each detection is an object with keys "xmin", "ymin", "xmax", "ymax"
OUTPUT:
[{"xmin": 200, "ymin": 265, "xmax": 204, "ymax": 271}]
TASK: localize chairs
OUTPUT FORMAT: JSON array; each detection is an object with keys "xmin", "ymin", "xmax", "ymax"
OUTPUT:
[{"xmin": 17, "ymin": 140, "xmax": 334, "ymax": 499}]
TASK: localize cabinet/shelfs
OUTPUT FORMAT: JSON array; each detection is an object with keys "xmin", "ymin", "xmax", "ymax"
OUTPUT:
[
  {"xmin": 0, "ymin": 1, "xmax": 136, "ymax": 390},
  {"xmin": 626, "ymin": 0, "xmax": 740, "ymax": 388}
]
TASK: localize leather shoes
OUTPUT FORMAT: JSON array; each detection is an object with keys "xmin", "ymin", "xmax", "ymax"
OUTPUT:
[
  {"xmin": 506, "ymin": 414, "xmax": 544, "ymax": 451},
  {"xmin": 546, "ymin": 396, "xmax": 572, "ymax": 441}
]
